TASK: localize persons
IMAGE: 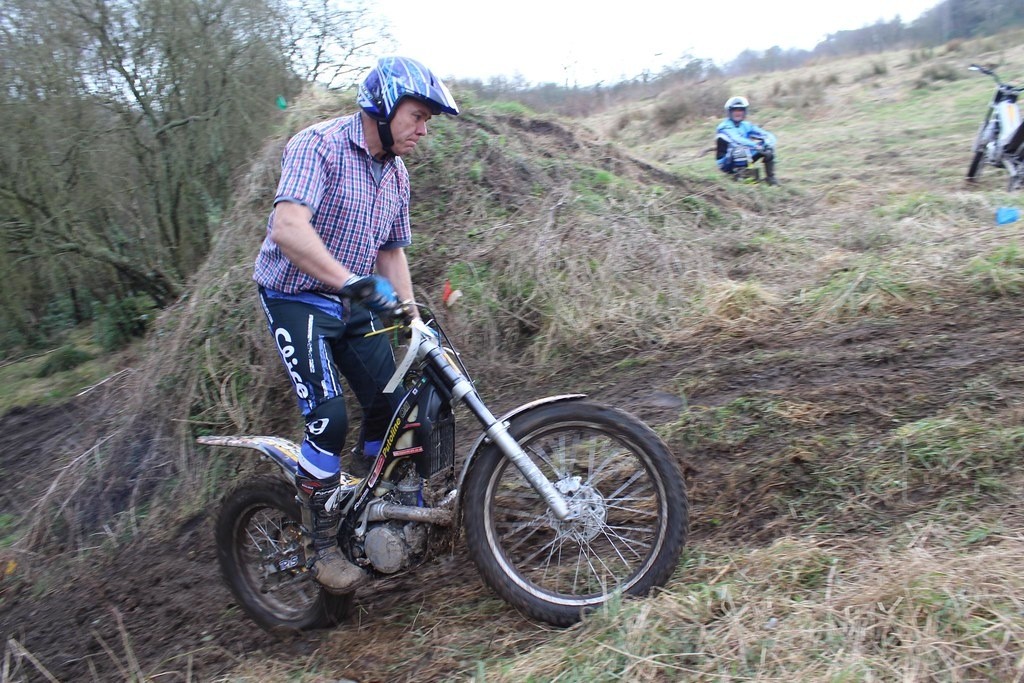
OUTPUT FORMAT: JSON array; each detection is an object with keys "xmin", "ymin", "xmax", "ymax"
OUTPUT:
[
  {"xmin": 715, "ymin": 96, "xmax": 778, "ymax": 187},
  {"xmin": 252, "ymin": 57, "xmax": 460, "ymax": 595}
]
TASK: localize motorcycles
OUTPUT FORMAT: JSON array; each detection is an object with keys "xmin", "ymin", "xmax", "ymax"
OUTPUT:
[
  {"xmin": 966, "ymin": 60, "xmax": 1024, "ymax": 191},
  {"xmin": 194, "ymin": 299, "xmax": 693, "ymax": 643}
]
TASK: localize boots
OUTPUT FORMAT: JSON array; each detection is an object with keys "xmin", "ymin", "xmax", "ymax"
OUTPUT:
[
  {"xmin": 350, "ymin": 449, "xmax": 374, "ymax": 479},
  {"xmin": 295, "ymin": 471, "xmax": 366, "ymax": 594},
  {"xmin": 765, "ymin": 162, "xmax": 779, "ymax": 186}
]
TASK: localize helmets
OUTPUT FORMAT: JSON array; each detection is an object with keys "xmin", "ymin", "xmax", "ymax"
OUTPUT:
[
  {"xmin": 724, "ymin": 97, "xmax": 747, "ymax": 112},
  {"xmin": 355, "ymin": 56, "xmax": 459, "ymax": 121}
]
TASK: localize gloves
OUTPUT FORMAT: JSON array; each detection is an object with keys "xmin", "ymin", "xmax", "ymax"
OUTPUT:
[
  {"xmin": 344, "ymin": 269, "xmax": 398, "ymax": 319},
  {"xmin": 403, "ymin": 317, "xmax": 441, "ymax": 346},
  {"xmin": 762, "ymin": 150, "xmax": 775, "ymax": 164}
]
